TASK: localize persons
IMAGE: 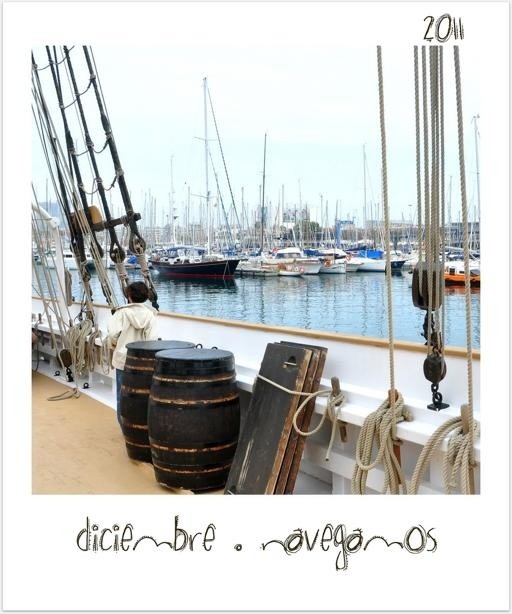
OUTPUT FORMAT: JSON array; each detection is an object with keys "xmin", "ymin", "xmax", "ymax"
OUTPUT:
[{"xmin": 107, "ymin": 280, "xmax": 158, "ymax": 436}]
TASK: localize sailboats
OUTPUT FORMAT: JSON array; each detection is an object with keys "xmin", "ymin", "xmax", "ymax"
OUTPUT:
[{"xmin": 33, "ymin": 77, "xmax": 480, "ymax": 285}]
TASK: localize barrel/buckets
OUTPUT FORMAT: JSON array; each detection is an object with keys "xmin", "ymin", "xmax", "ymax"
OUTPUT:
[
  {"xmin": 116, "ymin": 336, "xmax": 203, "ymax": 463},
  {"xmin": 146, "ymin": 346, "xmax": 241, "ymax": 493}
]
